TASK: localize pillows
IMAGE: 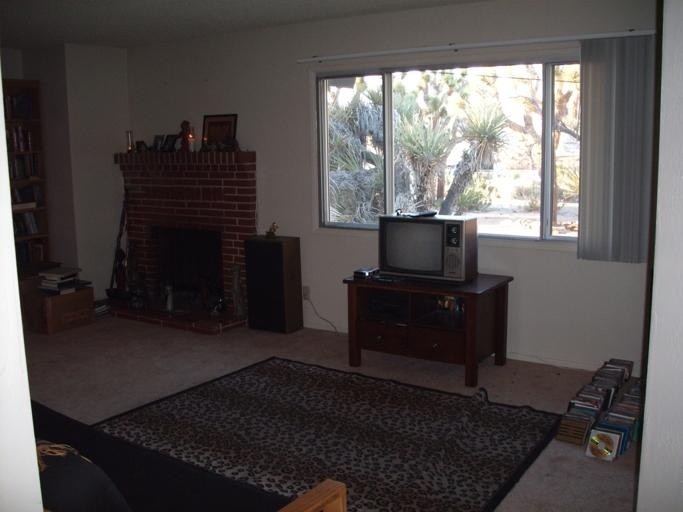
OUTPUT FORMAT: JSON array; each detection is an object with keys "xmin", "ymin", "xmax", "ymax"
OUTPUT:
[{"xmin": 37, "ymin": 438, "xmax": 124, "ymax": 511}]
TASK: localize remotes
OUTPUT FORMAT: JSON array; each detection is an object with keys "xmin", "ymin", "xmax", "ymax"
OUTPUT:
[{"xmin": 410, "ymin": 211, "xmax": 437, "ymax": 217}]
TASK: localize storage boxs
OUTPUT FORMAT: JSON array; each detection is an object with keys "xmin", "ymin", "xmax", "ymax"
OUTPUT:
[{"xmin": 20, "ymin": 281, "xmax": 95, "ymax": 336}]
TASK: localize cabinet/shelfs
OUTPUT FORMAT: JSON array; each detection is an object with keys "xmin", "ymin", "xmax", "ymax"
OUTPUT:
[
  {"xmin": 342, "ymin": 264, "xmax": 514, "ymax": 386},
  {"xmin": 2, "ymin": 76, "xmax": 51, "ymax": 280}
]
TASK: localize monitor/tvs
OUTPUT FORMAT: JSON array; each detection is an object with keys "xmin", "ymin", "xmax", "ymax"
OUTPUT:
[{"xmin": 378, "ymin": 213, "xmax": 477, "ymax": 285}]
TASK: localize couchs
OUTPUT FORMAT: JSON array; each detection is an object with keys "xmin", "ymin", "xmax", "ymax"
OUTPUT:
[{"xmin": 30, "ymin": 398, "xmax": 349, "ymax": 511}]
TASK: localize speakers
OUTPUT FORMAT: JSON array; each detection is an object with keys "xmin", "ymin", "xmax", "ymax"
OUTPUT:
[{"xmin": 245, "ymin": 236, "xmax": 303, "ymax": 333}]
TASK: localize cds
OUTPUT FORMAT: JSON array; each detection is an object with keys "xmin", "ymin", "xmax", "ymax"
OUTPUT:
[{"xmin": 589, "ymin": 433, "xmax": 613, "ymax": 457}]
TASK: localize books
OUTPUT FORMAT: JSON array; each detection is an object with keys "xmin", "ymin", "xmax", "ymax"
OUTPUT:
[{"xmin": 2, "ymin": 92, "xmax": 92, "ymax": 296}]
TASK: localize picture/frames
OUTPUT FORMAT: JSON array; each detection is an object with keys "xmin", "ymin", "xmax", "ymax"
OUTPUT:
[{"xmin": 202, "ymin": 114, "xmax": 237, "ymax": 143}]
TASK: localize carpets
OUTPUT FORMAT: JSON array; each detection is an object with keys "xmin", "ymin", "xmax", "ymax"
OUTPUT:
[{"xmin": 85, "ymin": 354, "xmax": 567, "ymax": 509}]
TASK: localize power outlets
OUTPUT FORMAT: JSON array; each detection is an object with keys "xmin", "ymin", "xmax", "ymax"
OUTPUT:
[{"xmin": 301, "ymin": 286, "xmax": 309, "ymax": 300}]
